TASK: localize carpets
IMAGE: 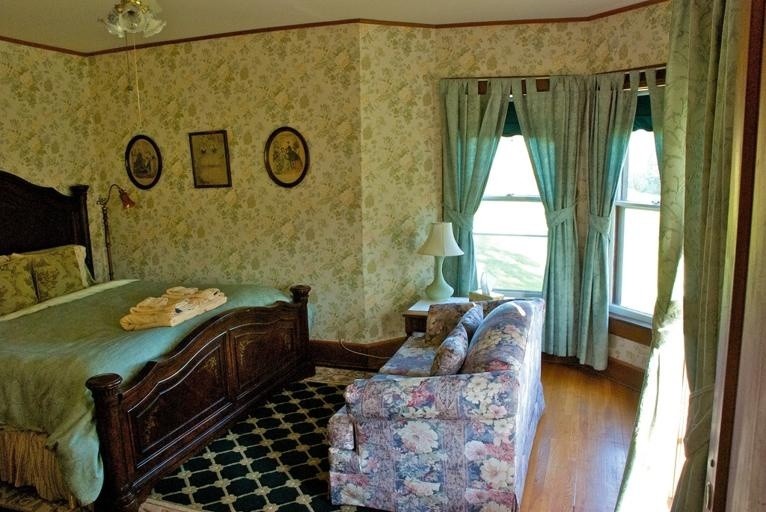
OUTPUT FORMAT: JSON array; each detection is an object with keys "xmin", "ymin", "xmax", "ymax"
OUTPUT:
[{"xmin": 145, "ymin": 379, "xmax": 347, "ymax": 511}]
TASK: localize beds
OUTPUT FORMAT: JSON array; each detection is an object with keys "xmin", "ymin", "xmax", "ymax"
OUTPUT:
[{"xmin": 0, "ymin": 169, "xmax": 311, "ymax": 511}]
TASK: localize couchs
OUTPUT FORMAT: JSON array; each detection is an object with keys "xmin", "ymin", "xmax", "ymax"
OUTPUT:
[{"xmin": 326, "ymin": 297, "xmax": 545, "ymax": 511}]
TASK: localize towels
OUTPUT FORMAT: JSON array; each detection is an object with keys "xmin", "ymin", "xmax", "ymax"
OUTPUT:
[{"xmin": 119, "ymin": 286, "xmax": 227, "ymax": 331}]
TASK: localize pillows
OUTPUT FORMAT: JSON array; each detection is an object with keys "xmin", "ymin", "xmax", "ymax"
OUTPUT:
[
  {"xmin": 430, "ymin": 304, "xmax": 483, "ymax": 375},
  {"xmin": 0, "ymin": 243, "xmax": 96, "ymax": 316}
]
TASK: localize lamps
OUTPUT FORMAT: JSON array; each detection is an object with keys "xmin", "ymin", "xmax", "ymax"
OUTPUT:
[
  {"xmin": 416, "ymin": 221, "xmax": 464, "ymax": 302},
  {"xmin": 97, "ymin": 1, "xmax": 166, "ymax": 39}
]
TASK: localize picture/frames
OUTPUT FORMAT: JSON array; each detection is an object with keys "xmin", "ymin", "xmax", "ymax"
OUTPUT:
[{"xmin": 125, "ymin": 126, "xmax": 310, "ymax": 190}]
